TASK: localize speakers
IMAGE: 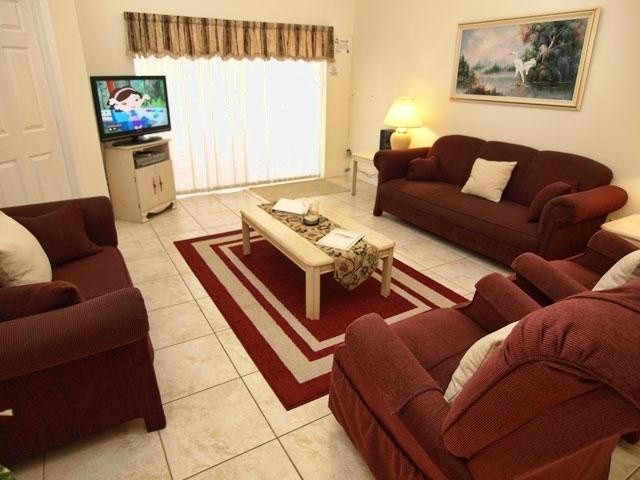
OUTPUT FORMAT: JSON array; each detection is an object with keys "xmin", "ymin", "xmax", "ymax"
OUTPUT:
[{"xmin": 380, "ymin": 129, "xmax": 395, "ymax": 150}]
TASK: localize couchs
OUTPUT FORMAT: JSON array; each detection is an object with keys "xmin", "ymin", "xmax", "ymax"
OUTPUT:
[{"xmin": 372, "ymin": 134, "xmax": 628, "ymax": 263}]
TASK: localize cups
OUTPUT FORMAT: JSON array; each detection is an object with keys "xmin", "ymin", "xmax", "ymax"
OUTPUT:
[{"xmin": 302, "ymin": 199, "xmax": 321, "ymax": 225}]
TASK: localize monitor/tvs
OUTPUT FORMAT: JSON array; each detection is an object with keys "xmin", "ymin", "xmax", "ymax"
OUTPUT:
[{"xmin": 90, "ymin": 75, "xmax": 171, "ymax": 146}]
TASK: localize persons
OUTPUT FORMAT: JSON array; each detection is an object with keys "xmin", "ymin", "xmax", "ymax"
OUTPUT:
[{"xmin": 105, "ymin": 86, "xmax": 149, "ymax": 130}]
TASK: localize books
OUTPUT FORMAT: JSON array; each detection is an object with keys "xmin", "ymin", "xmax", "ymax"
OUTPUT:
[{"xmin": 270, "ymin": 198, "xmax": 309, "ymax": 218}]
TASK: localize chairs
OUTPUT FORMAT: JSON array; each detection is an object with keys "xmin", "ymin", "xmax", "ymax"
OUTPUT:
[
  {"xmin": 328, "ymin": 273, "xmax": 640, "ymax": 479},
  {"xmin": 512, "ymin": 232, "xmax": 640, "ymax": 301},
  {"xmin": 0, "ymin": 194, "xmax": 165, "ymax": 468}
]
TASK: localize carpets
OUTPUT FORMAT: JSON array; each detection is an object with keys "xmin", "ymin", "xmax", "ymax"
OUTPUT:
[{"xmin": 172, "ymin": 226, "xmax": 467, "ymax": 411}]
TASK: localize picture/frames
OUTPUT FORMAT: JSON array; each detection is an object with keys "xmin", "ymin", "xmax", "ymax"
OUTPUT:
[{"xmin": 449, "ymin": 7, "xmax": 601, "ymax": 112}]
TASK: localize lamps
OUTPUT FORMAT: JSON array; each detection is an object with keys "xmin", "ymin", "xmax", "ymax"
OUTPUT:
[{"xmin": 383, "ymin": 96, "xmax": 423, "ymax": 150}]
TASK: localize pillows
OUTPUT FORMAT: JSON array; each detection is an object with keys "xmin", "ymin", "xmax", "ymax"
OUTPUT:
[
  {"xmin": 409, "ymin": 156, "xmax": 442, "ymax": 180},
  {"xmin": 0, "ymin": 280, "xmax": 83, "ymax": 321},
  {"xmin": 443, "ymin": 321, "xmax": 521, "ymax": 405},
  {"xmin": 527, "ymin": 182, "xmax": 572, "ymax": 223},
  {"xmin": 461, "ymin": 158, "xmax": 518, "ymax": 204},
  {"xmin": 592, "ymin": 248, "xmax": 640, "ymax": 291},
  {"xmin": 0, "ymin": 210, "xmax": 52, "ymax": 288},
  {"xmin": 15, "ymin": 201, "xmax": 103, "ymax": 269}
]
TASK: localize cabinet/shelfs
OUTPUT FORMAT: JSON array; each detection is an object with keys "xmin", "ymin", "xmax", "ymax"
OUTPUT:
[{"xmin": 104, "ymin": 136, "xmax": 176, "ymax": 223}]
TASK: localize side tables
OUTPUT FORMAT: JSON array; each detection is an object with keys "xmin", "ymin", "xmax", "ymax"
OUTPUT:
[
  {"xmin": 601, "ymin": 211, "xmax": 640, "ymax": 247},
  {"xmin": 351, "ymin": 153, "xmax": 374, "ymax": 196}
]
